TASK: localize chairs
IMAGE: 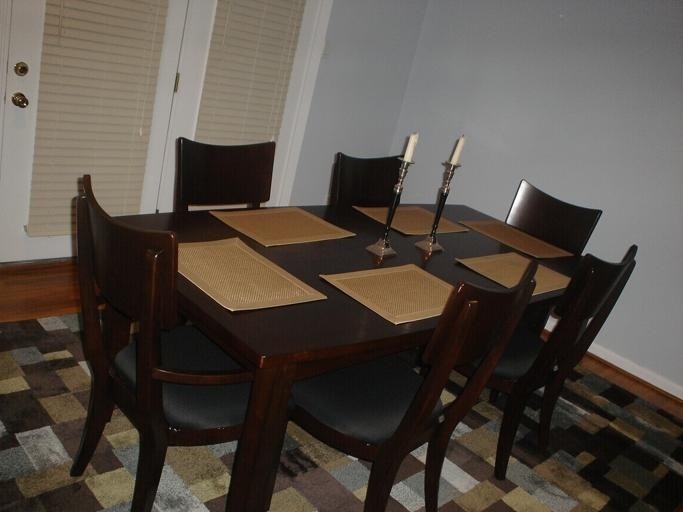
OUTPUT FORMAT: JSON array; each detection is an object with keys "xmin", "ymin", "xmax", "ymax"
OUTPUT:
[
  {"xmin": 328, "ymin": 151, "xmax": 404, "ymax": 207},
  {"xmin": 71, "ymin": 174, "xmax": 257, "ymax": 511},
  {"xmin": 503, "ymin": 180, "xmax": 603, "ymax": 255},
  {"xmin": 454, "ymin": 245, "xmax": 640, "ymax": 480},
  {"xmin": 171, "ymin": 134, "xmax": 278, "ymax": 212},
  {"xmin": 281, "ymin": 261, "xmax": 538, "ymax": 512}
]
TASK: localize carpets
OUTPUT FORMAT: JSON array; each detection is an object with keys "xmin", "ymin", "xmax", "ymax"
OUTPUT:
[{"xmin": 1, "ymin": 311, "xmax": 682, "ymax": 511}]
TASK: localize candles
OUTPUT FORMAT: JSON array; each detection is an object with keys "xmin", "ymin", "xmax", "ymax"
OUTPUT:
[
  {"xmin": 449, "ymin": 135, "xmax": 468, "ymax": 167},
  {"xmin": 403, "ymin": 128, "xmax": 421, "ymax": 164}
]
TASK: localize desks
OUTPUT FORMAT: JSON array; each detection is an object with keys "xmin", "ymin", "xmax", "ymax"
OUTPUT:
[{"xmin": 100, "ymin": 204, "xmax": 619, "ymax": 512}]
